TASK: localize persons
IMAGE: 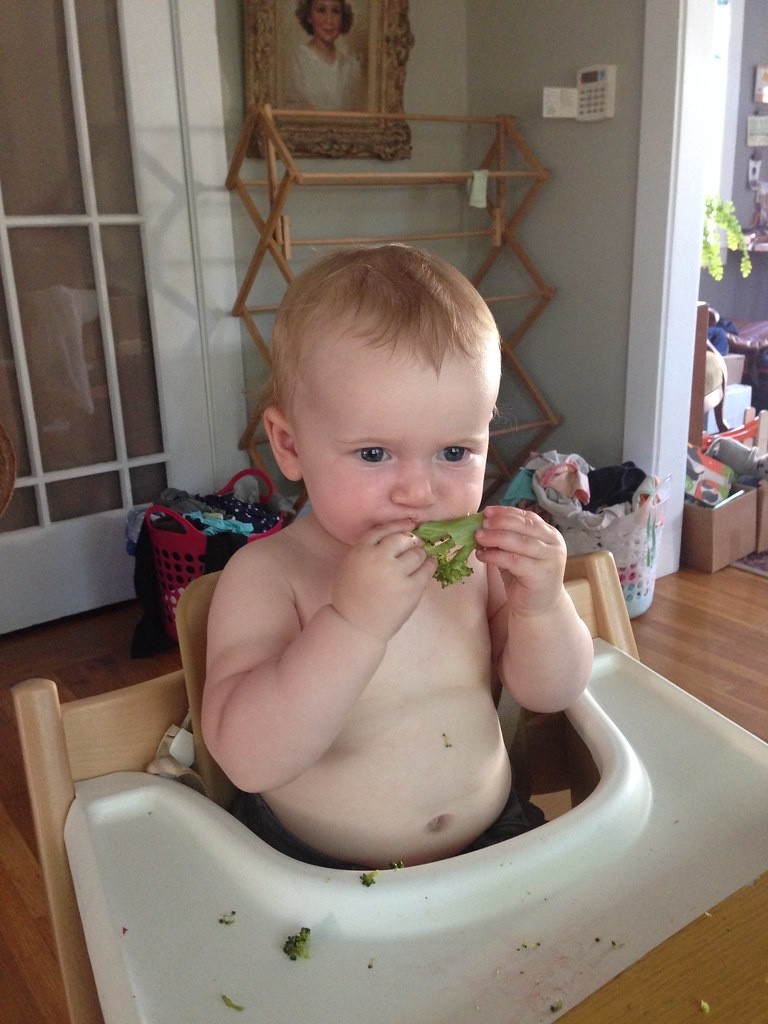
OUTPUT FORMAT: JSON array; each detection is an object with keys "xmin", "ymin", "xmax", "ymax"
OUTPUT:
[{"xmin": 199, "ymin": 241, "xmax": 596, "ymax": 874}]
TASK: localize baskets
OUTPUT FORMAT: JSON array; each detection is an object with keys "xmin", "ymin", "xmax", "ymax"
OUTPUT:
[
  {"xmin": 145, "ymin": 469, "xmax": 283, "ymax": 642},
  {"xmin": 548, "ymin": 474, "xmax": 673, "ymax": 619}
]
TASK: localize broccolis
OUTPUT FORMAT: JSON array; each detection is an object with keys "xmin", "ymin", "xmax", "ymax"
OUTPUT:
[
  {"xmin": 412, "ymin": 510, "xmax": 485, "ymax": 588},
  {"xmin": 283, "ymin": 927, "xmax": 310, "ymax": 961}
]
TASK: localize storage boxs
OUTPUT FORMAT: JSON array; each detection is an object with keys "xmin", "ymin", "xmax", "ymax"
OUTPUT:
[{"xmin": 680, "ymin": 354, "xmax": 768, "ymax": 574}]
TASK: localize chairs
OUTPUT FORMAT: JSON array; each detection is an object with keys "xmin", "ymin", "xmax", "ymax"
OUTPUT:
[{"xmin": 11, "ymin": 551, "xmax": 643, "ymax": 1023}]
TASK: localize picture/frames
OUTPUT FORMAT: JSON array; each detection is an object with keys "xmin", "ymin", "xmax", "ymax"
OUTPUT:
[{"xmin": 242, "ymin": 0, "xmax": 416, "ymax": 160}]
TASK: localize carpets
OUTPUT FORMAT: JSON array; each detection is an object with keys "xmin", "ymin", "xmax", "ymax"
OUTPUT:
[{"xmin": 730, "ymin": 550, "xmax": 768, "ymax": 578}]
